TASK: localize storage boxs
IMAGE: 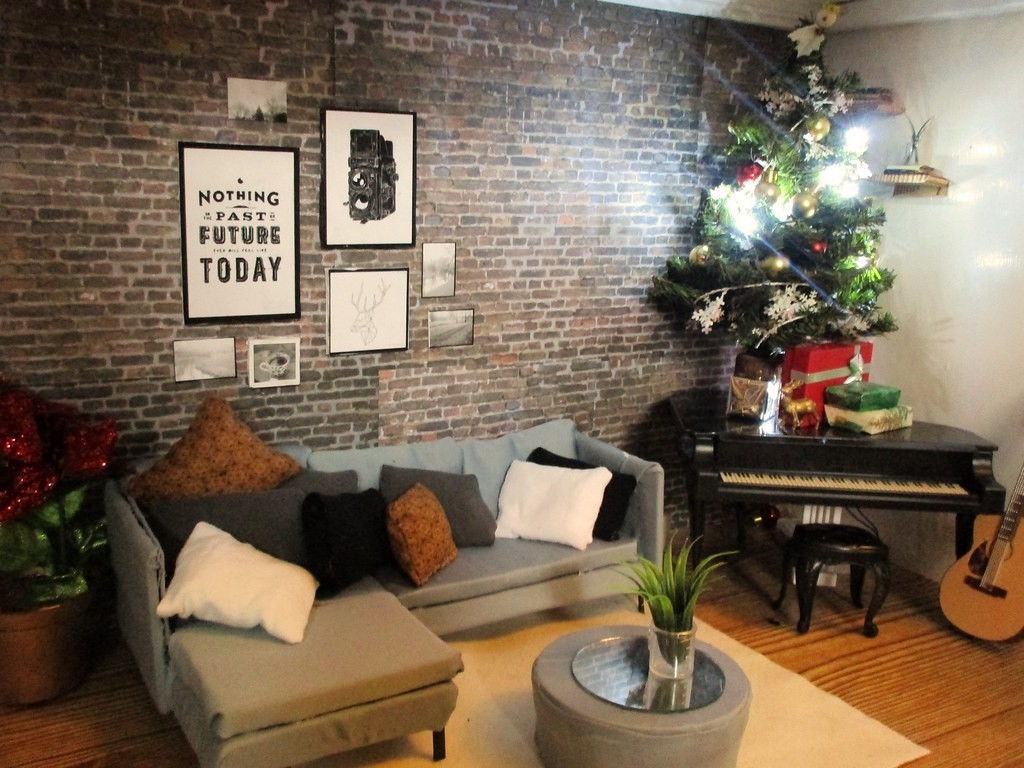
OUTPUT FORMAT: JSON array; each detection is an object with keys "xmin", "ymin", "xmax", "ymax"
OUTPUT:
[
  {"xmin": 778, "ymin": 341, "xmax": 873, "ymax": 430},
  {"xmin": 825, "ymin": 380, "xmax": 901, "ymax": 412},
  {"xmin": 822, "ymin": 404, "xmax": 913, "ymax": 436},
  {"xmin": 723, "ymin": 375, "xmax": 768, "ymax": 419}
]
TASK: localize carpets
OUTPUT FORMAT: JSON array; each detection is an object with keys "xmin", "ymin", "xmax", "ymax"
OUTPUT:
[{"xmin": 294, "ymin": 591, "xmax": 933, "ymax": 768}]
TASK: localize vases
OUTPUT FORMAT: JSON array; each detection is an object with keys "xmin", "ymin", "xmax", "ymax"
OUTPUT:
[{"xmin": 0, "ymin": 601, "xmax": 86, "ymax": 708}]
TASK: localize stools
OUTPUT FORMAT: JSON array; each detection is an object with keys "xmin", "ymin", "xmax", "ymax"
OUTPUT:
[{"xmin": 767, "ymin": 523, "xmax": 893, "ymax": 639}]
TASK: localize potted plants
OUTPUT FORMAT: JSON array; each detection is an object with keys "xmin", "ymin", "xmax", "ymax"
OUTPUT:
[{"xmin": 597, "ymin": 530, "xmax": 739, "ymax": 682}]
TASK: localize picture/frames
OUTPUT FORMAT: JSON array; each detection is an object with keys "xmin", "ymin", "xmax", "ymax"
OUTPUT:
[
  {"xmin": 325, "ymin": 267, "xmax": 409, "ymax": 355},
  {"xmin": 318, "ymin": 105, "xmax": 418, "ymax": 251},
  {"xmin": 248, "ymin": 336, "xmax": 301, "ymax": 388},
  {"xmin": 177, "ymin": 141, "xmax": 300, "ymax": 325}
]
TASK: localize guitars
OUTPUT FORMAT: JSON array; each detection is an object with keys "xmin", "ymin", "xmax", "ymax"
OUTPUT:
[{"xmin": 939, "ymin": 458, "xmax": 1024, "ymax": 645}]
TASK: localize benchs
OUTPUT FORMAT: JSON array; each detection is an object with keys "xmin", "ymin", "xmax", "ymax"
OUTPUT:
[
  {"xmin": 307, "ymin": 417, "xmax": 664, "ymax": 637},
  {"xmin": 103, "ymin": 440, "xmax": 464, "ymax": 768}
]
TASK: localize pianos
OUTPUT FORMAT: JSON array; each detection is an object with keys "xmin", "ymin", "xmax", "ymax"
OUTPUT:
[{"xmin": 668, "ymin": 388, "xmax": 1006, "ymax": 577}]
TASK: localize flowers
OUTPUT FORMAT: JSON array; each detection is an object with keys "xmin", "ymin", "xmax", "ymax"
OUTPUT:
[{"xmin": 0, "ymin": 374, "xmax": 119, "ymax": 608}]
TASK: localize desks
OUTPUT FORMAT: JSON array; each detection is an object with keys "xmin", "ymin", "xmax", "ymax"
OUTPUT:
[{"xmin": 530, "ymin": 624, "xmax": 752, "ymax": 768}]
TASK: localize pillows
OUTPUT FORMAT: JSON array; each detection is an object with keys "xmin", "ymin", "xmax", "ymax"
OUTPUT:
[
  {"xmin": 278, "ymin": 467, "xmax": 358, "ymax": 498},
  {"xmin": 154, "ymin": 521, "xmax": 320, "ymax": 646},
  {"xmin": 379, "ymin": 464, "xmax": 497, "ymax": 548},
  {"xmin": 131, "ymin": 395, "xmax": 301, "ymax": 497},
  {"xmin": 135, "ymin": 488, "xmax": 305, "ymax": 566},
  {"xmin": 302, "ymin": 488, "xmax": 392, "ymax": 602},
  {"xmin": 386, "ymin": 480, "xmax": 459, "ymax": 587},
  {"xmin": 491, "ymin": 459, "xmax": 613, "ymax": 552},
  {"xmin": 525, "ymin": 446, "xmax": 638, "ymax": 542}
]
